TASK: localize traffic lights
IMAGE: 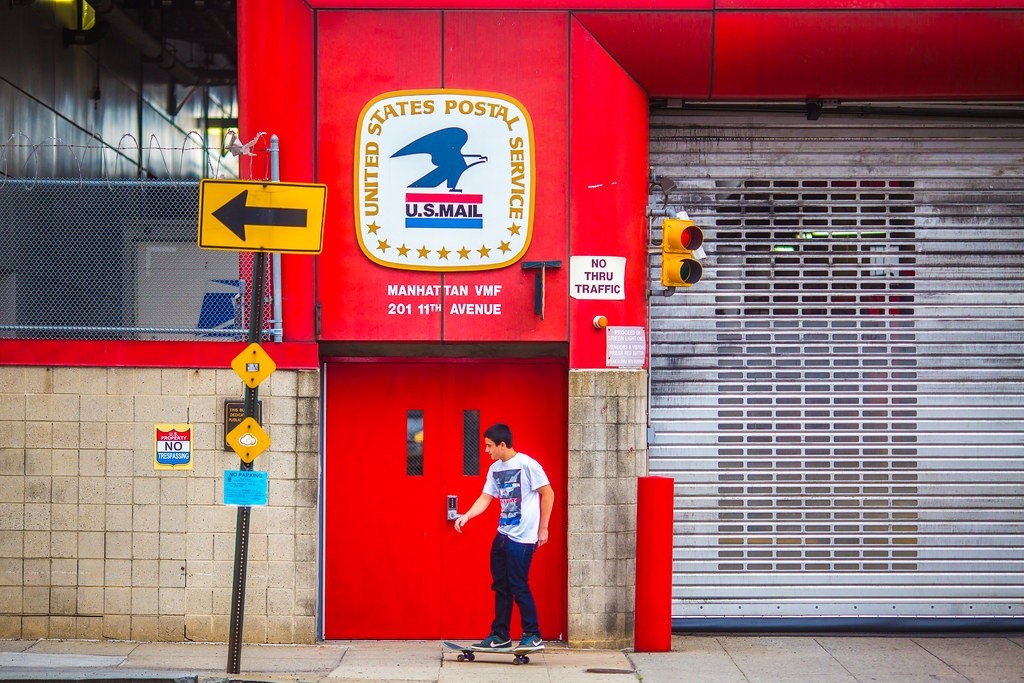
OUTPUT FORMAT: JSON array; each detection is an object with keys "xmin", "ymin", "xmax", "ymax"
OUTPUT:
[{"xmin": 662, "ymin": 217, "xmax": 703, "ymax": 288}]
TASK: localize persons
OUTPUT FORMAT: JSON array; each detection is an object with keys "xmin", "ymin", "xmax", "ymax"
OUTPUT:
[{"xmin": 454, "ymin": 423, "xmax": 555, "ymax": 651}]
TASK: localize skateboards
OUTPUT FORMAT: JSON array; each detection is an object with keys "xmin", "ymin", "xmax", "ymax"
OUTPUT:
[{"xmin": 443, "ymin": 641, "xmax": 545, "ymax": 665}]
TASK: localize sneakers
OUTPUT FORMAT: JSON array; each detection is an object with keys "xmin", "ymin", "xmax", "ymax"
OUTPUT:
[
  {"xmin": 470, "ymin": 634, "xmax": 511, "ymax": 649},
  {"xmin": 515, "ymin": 632, "xmax": 545, "ymax": 651}
]
